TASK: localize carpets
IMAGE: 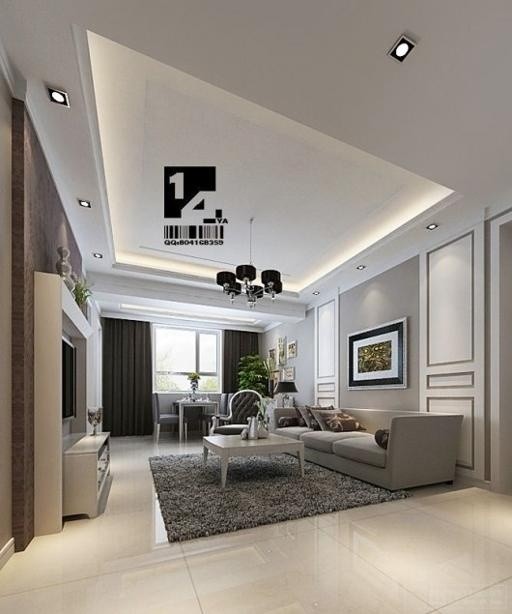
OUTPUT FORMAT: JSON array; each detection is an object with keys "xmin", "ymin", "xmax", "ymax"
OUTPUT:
[{"xmin": 148, "ymin": 451, "xmax": 412, "ymax": 543}]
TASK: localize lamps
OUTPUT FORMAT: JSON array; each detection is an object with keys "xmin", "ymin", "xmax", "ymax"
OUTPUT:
[
  {"xmin": 272, "ymin": 381, "xmax": 299, "ymax": 407},
  {"xmin": 216, "ymin": 217, "xmax": 283, "ymax": 310}
]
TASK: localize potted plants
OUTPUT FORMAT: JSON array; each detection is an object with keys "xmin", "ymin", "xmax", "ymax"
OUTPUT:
[{"xmin": 187, "ymin": 371, "xmax": 200, "ymax": 402}]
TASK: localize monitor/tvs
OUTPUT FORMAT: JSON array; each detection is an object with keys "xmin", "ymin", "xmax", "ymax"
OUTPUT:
[{"xmin": 61, "ymin": 336, "xmax": 77, "ymax": 422}]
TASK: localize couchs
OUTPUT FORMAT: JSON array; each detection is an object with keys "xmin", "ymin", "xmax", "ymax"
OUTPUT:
[{"xmin": 267, "ymin": 407, "xmax": 464, "ymax": 492}]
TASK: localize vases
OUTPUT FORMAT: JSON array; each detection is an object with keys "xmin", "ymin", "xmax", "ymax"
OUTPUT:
[{"xmin": 56, "ymin": 246, "xmax": 75, "ymax": 292}]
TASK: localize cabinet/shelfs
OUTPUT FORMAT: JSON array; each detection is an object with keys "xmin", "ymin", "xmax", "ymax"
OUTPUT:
[{"xmin": 64, "ymin": 430, "xmax": 112, "ymax": 519}]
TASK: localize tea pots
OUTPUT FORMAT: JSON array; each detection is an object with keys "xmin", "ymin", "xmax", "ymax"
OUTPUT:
[{"xmin": 241, "ymin": 414, "xmax": 268, "ymax": 439}]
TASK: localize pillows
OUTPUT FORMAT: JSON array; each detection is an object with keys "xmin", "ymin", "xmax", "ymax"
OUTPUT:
[
  {"xmin": 374, "ymin": 428, "xmax": 390, "ymax": 449},
  {"xmin": 278, "ymin": 404, "xmax": 367, "ymax": 433}
]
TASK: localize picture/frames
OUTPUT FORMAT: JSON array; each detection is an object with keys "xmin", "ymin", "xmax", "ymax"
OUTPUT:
[
  {"xmin": 346, "ymin": 315, "xmax": 409, "ymax": 390},
  {"xmin": 264, "ymin": 340, "xmax": 298, "ymax": 387}
]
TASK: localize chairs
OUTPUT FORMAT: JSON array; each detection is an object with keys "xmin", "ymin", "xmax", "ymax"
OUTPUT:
[
  {"xmin": 198, "ymin": 393, "xmax": 235, "ymax": 436},
  {"xmin": 209, "ymin": 389, "xmax": 263, "ymax": 435},
  {"xmin": 152, "ymin": 393, "xmax": 188, "ymax": 442}
]
